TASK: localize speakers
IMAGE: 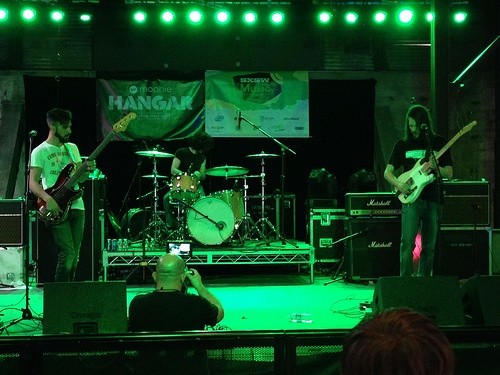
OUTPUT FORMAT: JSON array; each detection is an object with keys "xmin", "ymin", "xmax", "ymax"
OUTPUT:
[
  {"xmin": 36, "ymin": 179, "xmax": 108, "ymax": 287},
  {"xmin": 429, "ymin": 226, "xmax": 492, "ymax": 285},
  {"xmin": 43, "ymin": 281, "xmax": 127, "ymax": 334},
  {"xmin": 370, "ymin": 275, "xmax": 467, "ymax": 327},
  {"xmin": 461, "ymin": 274, "xmax": 500, "ymax": 327},
  {"xmin": 434, "ymin": 181, "xmax": 492, "ymax": 227},
  {"xmin": 343, "ymin": 218, "xmax": 400, "ymax": 282},
  {"xmin": 0, "ymin": 198, "xmax": 28, "ymax": 245}
]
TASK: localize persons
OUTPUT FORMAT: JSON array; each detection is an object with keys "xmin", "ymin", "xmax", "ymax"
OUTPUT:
[
  {"xmin": 129, "ymin": 253, "xmax": 223, "ymax": 375},
  {"xmin": 164, "ymin": 135, "xmax": 210, "ymax": 239},
  {"xmin": 384, "ymin": 106, "xmax": 453, "ymax": 276},
  {"xmin": 28, "ymin": 108, "xmax": 96, "ymax": 281},
  {"xmin": 342, "ymin": 308, "xmax": 454, "ymax": 375}
]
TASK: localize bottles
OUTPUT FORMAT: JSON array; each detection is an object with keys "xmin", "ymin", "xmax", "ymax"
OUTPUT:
[
  {"xmin": 117, "ymin": 239, "xmax": 123, "ymax": 251},
  {"xmin": 123, "ymin": 239, "xmax": 128, "ymax": 251}
]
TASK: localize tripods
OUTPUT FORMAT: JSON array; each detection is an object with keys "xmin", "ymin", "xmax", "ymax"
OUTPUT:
[
  {"xmin": 227, "ymin": 157, "xmax": 300, "ymax": 249},
  {"xmin": 128, "ymin": 153, "xmax": 173, "ymax": 252}
]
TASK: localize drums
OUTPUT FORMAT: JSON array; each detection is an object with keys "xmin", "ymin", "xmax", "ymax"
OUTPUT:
[
  {"xmin": 210, "ymin": 190, "xmax": 244, "ymax": 224},
  {"xmin": 120, "ymin": 207, "xmax": 153, "ymax": 242},
  {"xmin": 186, "ymin": 196, "xmax": 236, "ymax": 246},
  {"xmin": 170, "ymin": 172, "xmax": 200, "ymax": 201}
]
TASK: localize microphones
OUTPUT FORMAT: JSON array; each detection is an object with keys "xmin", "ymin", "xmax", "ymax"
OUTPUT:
[
  {"xmin": 421, "ymin": 123, "xmax": 428, "ymax": 129},
  {"xmin": 235, "ymin": 108, "xmax": 242, "ymax": 130},
  {"xmin": 29, "ymin": 130, "xmax": 37, "ymax": 137}
]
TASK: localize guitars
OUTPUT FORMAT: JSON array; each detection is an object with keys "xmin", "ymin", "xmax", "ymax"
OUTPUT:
[
  {"xmin": 36, "ymin": 112, "xmax": 138, "ymax": 225},
  {"xmin": 394, "ymin": 120, "xmax": 478, "ymax": 205}
]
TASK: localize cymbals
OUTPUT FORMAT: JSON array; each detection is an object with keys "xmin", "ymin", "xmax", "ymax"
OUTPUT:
[
  {"xmin": 246, "ymin": 152, "xmax": 280, "ymax": 158},
  {"xmin": 135, "ymin": 150, "xmax": 175, "ymax": 158},
  {"xmin": 205, "ymin": 165, "xmax": 249, "ymax": 177},
  {"xmin": 142, "ymin": 174, "xmax": 168, "ymax": 178}
]
